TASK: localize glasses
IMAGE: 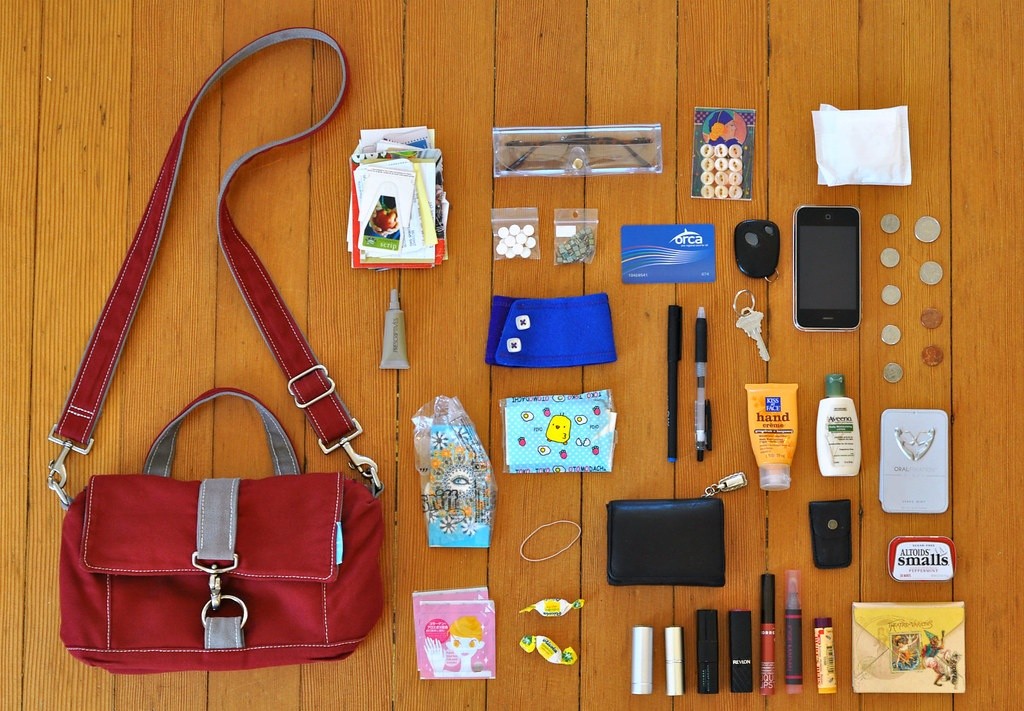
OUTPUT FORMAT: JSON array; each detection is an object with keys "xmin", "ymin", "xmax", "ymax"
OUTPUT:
[{"xmin": 505, "ymin": 133, "xmax": 654, "ymax": 169}]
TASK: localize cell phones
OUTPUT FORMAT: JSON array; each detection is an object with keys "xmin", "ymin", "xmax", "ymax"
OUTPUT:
[{"xmin": 791, "ymin": 204, "xmax": 864, "ymax": 333}]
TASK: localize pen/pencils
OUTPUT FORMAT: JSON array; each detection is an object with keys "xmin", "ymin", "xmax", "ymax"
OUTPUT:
[
  {"xmin": 666, "ymin": 304, "xmax": 683, "ymax": 463},
  {"xmin": 693, "ymin": 306, "xmax": 713, "ymax": 462}
]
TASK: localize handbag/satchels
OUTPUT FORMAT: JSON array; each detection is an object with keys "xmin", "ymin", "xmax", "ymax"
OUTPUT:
[{"xmin": 44, "ymin": 26, "xmax": 387, "ymax": 675}]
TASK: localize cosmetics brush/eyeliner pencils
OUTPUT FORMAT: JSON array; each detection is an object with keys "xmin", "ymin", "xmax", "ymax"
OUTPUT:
[
  {"xmin": 760, "ymin": 569, "xmax": 778, "ymax": 699},
  {"xmin": 784, "ymin": 568, "xmax": 807, "ymax": 695}
]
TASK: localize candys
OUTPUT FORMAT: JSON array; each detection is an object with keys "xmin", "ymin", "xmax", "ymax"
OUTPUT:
[
  {"xmin": 519, "ymin": 635, "xmax": 578, "ymax": 665},
  {"xmin": 519, "ymin": 598, "xmax": 586, "ymax": 617}
]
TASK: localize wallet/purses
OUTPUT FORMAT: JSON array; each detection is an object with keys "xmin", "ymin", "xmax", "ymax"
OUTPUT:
[{"xmin": 606, "ymin": 498, "xmax": 727, "ymax": 588}]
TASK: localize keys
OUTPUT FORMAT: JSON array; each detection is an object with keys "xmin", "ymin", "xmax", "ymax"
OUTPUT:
[{"xmin": 731, "ymin": 288, "xmax": 773, "ymax": 362}]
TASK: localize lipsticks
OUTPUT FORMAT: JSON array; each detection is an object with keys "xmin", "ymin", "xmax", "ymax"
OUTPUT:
[
  {"xmin": 810, "ymin": 614, "xmax": 838, "ymax": 696},
  {"xmin": 628, "ymin": 606, "xmax": 756, "ymax": 696}
]
